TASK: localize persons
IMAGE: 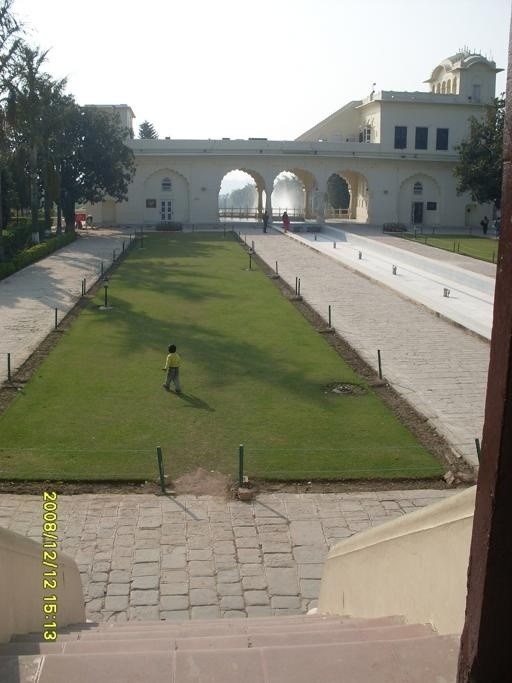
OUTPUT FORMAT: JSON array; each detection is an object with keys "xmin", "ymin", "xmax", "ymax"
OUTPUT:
[
  {"xmin": 282, "ymin": 212, "xmax": 290, "ymax": 233},
  {"xmin": 494, "ymin": 217, "xmax": 501, "ymax": 239},
  {"xmin": 480, "ymin": 216, "xmax": 490, "ymax": 234},
  {"xmin": 161, "ymin": 344, "xmax": 182, "ymax": 393},
  {"xmin": 263, "ymin": 210, "xmax": 269, "ymax": 233}
]
